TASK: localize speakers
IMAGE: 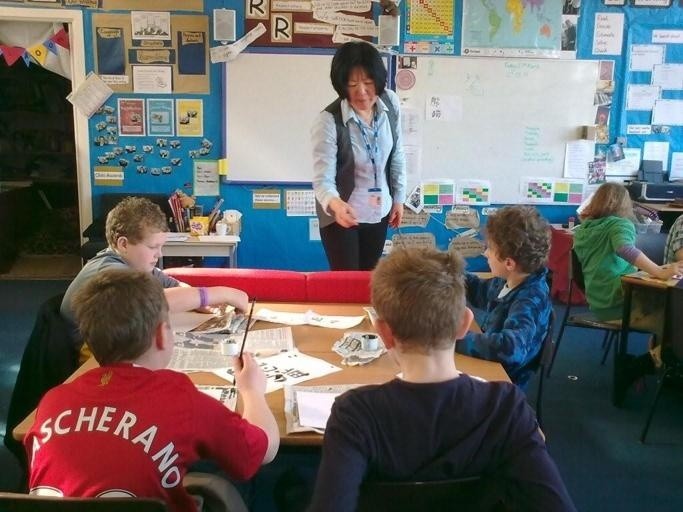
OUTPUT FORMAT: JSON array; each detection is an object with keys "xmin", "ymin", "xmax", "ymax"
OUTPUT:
[
  {"xmin": 213, "ymin": 7, "xmax": 236, "ymax": 45},
  {"xmin": 378, "ymin": 15, "xmax": 400, "ymax": 49}
]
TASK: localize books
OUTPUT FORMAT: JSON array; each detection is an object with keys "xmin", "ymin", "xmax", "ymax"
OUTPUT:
[{"xmin": 168, "ymin": 190, "xmax": 188, "ymax": 232}]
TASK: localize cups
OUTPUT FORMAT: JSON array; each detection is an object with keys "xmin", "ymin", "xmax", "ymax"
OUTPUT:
[
  {"xmin": 215, "ymin": 223, "xmax": 230, "ymax": 236},
  {"xmin": 360, "ymin": 333, "xmax": 381, "ymax": 352}
]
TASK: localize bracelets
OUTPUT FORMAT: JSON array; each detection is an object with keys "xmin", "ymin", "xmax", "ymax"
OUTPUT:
[{"xmin": 197, "ymin": 287, "xmax": 210, "ymax": 309}]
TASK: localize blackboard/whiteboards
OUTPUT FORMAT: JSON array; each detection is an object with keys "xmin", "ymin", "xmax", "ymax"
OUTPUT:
[{"xmin": 396, "ymin": 54, "xmax": 616, "ymax": 205}]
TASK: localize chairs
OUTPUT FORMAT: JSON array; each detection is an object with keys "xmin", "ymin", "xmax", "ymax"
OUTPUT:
[
  {"xmin": 357, "ymin": 474, "xmax": 504, "ymax": 512},
  {"xmin": 544, "ymin": 248, "xmax": 629, "ymax": 378},
  {"xmin": 6, "ymin": 290, "xmax": 81, "ymax": 430},
  {"xmin": 637, "ymin": 286, "xmax": 682, "ymax": 443},
  {"xmin": 513, "ymin": 309, "xmax": 557, "ymax": 430},
  {"xmin": 1, "ymin": 492, "xmax": 172, "ymax": 512}
]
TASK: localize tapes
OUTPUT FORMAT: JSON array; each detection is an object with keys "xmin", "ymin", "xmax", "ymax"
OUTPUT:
[{"xmin": 361, "ymin": 334, "xmax": 378, "ymax": 351}]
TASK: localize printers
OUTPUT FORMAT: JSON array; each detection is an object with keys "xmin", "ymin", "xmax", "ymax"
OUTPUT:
[{"xmin": 625, "ymin": 160, "xmax": 683, "ymax": 204}]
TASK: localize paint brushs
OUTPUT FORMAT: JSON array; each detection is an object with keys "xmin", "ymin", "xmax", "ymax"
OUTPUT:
[
  {"xmin": 220, "ymin": 47, "xmax": 392, "ymax": 190},
  {"xmin": 230, "ymin": 298, "xmax": 256, "ymax": 386}
]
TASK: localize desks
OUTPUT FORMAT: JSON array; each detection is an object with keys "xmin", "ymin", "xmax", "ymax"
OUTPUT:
[
  {"xmin": 638, "ymin": 200, "xmax": 682, "ymax": 214},
  {"xmin": 160, "ymin": 230, "xmax": 239, "ymax": 267},
  {"xmin": 616, "ymin": 260, "xmax": 683, "ymax": 406},
  {"xmin": 12, "ymin": 302, "xmax": 550, "ymax": 450}
]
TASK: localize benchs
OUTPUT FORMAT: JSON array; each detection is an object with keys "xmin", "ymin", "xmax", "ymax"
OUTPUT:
[{"xmin": 164, "ymin": 266, "xmax": 374, "ymax": 303}]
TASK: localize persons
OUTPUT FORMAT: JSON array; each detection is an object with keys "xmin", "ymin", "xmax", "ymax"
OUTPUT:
[
  {"xmin": 308, "ymin": 245, "xmax": 576, "ymax": 511},
  {"xmin": 662, "ymin": 211, "xmax": 683, "ymax": 268},
  {"xmin": 21, "ymin": 268, "xmax": 282, "ymax": 511},
  {"xmin": 572, "ymin": 182, "xmax": 682, "ymax": 408},
  {"xmin": 454, "ymin": 206, "xmax": 555, "ymax": 392},
  {"xmin": 588, "ymin": 161, "xmax": 607, "ymax": 185},
  {"xmin": 407, "ymin": 188, "xmax": 421, "ymax": 208},
  {"xmin": 94, "ymin": 105, "xmax": 212, "ymax": 175},
  {"xmin": 309, "ymin": 41, "xmax": 407, "ymax": 270},
  {"xmin": 57, "ymin": 196, "xmax": 250, "ymax": 370}
]
